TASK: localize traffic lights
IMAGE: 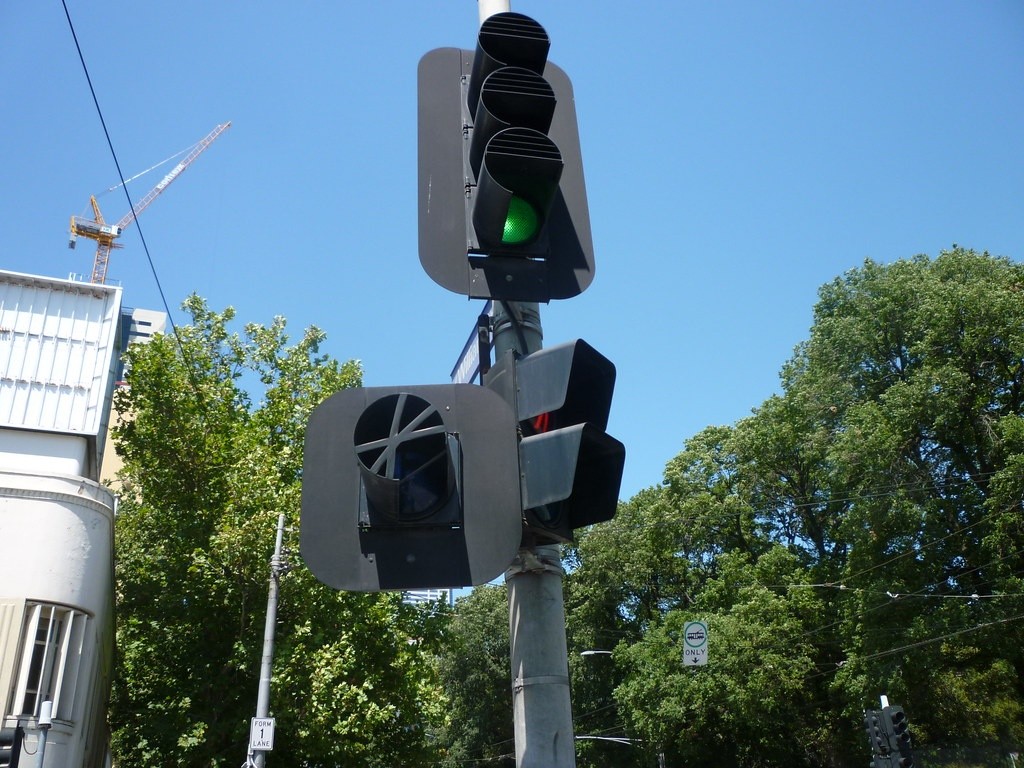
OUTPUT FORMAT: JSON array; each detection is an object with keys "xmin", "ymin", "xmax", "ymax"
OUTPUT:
[
  {"xmin": 870, "ymin": 754, "xmax": 891, "ymax": 768},
  {"xmin": 863, "ymin": 711, "xmax": 890, "ymax": 755},
  {"xmin": 481, "ymin": 337, "xmax": 626, "ymax": 547},
  {"xmin": 417, "ymin": 13, "xmax": 595, "ymax": 305},
  {"xmin": 883, "ymin": 706, "xmax": 912, "ymax": 751},
  {"xmin": 299, "ymin": 383, "xmax": 523, "ymax": 593},
  {"xmin": 891, "ymin": 750, "xmax": 916, "ymax": 768}
]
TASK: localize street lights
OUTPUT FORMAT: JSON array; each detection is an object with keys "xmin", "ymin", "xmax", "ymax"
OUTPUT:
[{"xmin": 580, "ymin": 650, "xmax": 665, "ymax": 768}]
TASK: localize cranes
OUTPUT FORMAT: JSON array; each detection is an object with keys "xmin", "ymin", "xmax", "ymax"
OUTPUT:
[{"xmin": 67, "ymin": 121, "xmax": 232, "ymax": 286}]
